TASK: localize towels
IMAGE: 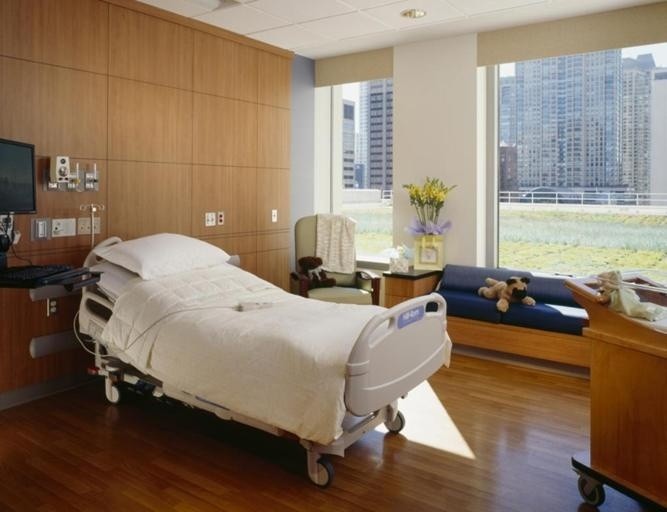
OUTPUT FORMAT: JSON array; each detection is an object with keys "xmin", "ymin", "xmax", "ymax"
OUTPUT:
[
  {"xmin": 608, "ymin": 287, "xmax": 665, "ymax": 323},
  {"xmin": 316, "ymin": 213, "xmax": 358, "ymax": 274}
]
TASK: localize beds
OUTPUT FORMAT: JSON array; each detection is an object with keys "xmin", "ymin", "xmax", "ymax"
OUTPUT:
[{"xmin": 78, "ymin": 236, "xmax": 453, "ymax": 489}]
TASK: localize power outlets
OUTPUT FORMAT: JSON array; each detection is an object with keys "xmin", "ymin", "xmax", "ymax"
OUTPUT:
[{"xmin": 46, "ymin": 296, "xmax": 60, "ymax": 317}]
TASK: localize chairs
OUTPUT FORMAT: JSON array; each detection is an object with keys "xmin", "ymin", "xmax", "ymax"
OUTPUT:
[{"xmin": 289, "ymin": 214, "xmax": 382, "ymax": 306}]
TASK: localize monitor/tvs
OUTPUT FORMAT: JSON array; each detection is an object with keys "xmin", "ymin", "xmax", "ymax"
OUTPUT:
[{"xmin": 0, "ymin": 138, "xmax": 37, "ymax": 215}]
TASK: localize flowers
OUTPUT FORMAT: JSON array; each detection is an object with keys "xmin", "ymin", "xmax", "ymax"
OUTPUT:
[{"xmin": 401, "ymin": 176, "xmax": 459, "ymax": 236}]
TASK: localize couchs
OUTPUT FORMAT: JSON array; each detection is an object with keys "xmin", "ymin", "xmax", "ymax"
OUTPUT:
[{"xmin": 423, "ymin": 264, "xmax": 593, "ymax": 381}]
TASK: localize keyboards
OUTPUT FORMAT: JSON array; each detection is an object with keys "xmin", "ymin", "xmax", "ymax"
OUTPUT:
[{"xmin": 0, "ymin": 263, "xmax": 73, "ymax": 280}]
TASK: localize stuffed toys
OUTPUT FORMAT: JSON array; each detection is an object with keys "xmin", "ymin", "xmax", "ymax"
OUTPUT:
[{"xmin": 475, "ymin": 276, "xmax": 535, "ymax": 312}]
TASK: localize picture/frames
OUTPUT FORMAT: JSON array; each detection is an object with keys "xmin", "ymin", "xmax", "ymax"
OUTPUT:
[{"xmin": 413, "ymin": 242, "xmax": 444, "ymax": 271}]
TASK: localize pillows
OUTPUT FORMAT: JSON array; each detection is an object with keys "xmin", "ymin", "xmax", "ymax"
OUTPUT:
[{"xmin": 91, "ymin": 232, "xmax": 233, "ymax": 281}]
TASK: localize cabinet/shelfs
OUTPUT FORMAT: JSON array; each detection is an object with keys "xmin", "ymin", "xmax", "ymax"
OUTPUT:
[{"xmin": 382, "ymin": 266, "xmax": 442, "ymax": 308}]
